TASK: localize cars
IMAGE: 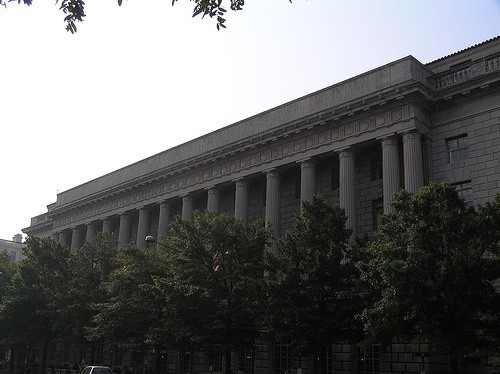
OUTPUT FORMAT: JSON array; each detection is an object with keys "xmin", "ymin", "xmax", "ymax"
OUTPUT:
[{"xmin": 81, "ymin": 366, "xmax": 114, "ymax": 374}]
